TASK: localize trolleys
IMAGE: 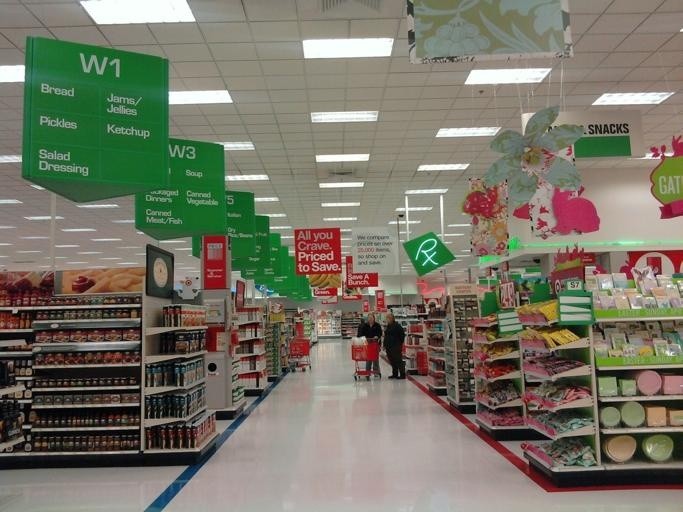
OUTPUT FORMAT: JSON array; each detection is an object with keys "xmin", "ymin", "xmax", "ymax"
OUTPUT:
[
  {"xmin": 288, "ymin": 338, "xmax": 311, "ymax": 372},
  {"xmin": 352, "ymin": 338, "xmax": 382, "ymax": 381}
]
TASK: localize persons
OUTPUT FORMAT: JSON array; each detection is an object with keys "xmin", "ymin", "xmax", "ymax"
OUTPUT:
[
  {"xmin": 381, "ymin": 313, "xmax": 407, "ymax": 378},
  {"xmin": 357, "ymin": 319, "xmax": 367, "ymax": 337},
  {"xmin": 363, "ymin": 313, "xmax": 382, "ymax": 380}
]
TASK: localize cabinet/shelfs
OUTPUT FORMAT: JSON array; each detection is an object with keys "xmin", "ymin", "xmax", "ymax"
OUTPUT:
[
  {"xmin": 0, "ymin": 269, "xmax": 220, "ymax": 463},
  {"xmin": 234, "ymin": 305, "xmax": 267, "ymax": 396},
  {"xmin": 303, "ymin": 314, "xmax": 313, "ymax": 348},
  {"xmin": 472, "ymin": 307, "xmax": 550, "ymax": 440},
  {"xmin": 263, "ymin": 305, "xmax": 284, "ymax": 382},
  {"xmin": 202, "ymin": 290, "xmax": 247, "ymax": 419},
  {"xmin": 520, "ymin": 318, "xmax": 682, "ymax": 482},
  {"xmin": 393, "ymin": 313, "xmax": 432, "ymax": 375},
  {"xmin": 279, "ymin": 317, "xmax": 294, "ymax": 372},
  {"xmin": 313, "ymin": 321, "xmax": 318, "ymax": 344},
  {"xmin": 423, "ymin": 319, "xmax": 447, "ymax": 396},
  {"xmin": 441, "ymin": 294, "xmax": 485, "ymax": 414}
]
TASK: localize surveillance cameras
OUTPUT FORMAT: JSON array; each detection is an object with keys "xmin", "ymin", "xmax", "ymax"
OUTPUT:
[{"xmin": 398, "ymin": 214, "xmax": 404, "ymax": 217}]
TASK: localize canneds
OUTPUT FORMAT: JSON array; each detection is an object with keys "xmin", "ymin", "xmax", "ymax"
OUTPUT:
[
  {"xmin": 33, "ymin": 376, "xmax": 137, "ymax": 387},
  {"xmin": 36, "ymin": 295, "xmax": 142, "ymax": 306},
  {"xmin": 35, "ymin": 308, "xmax": 142, "ymax": 319}
]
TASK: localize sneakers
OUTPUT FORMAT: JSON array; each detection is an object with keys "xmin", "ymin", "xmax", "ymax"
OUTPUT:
[{"xmin": 388, "ymin": 375, "xmax": 406, "ymax": 379}]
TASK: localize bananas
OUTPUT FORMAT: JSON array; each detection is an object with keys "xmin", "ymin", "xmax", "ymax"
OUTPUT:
[{"xmin": 309, "ymin": 273, "xmax": 341, "ymax": 289}]
